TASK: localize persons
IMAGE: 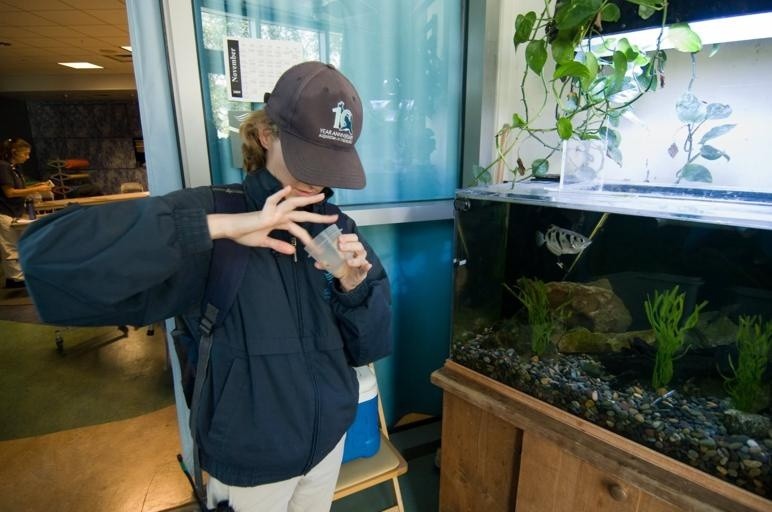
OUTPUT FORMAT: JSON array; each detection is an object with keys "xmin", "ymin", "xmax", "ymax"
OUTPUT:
[
  {"xmin": 20, "ymin": 60, "xmax": 391, "ymax": 512},
  {"xmin": 0, "ymin": 137, "xmax": 53, "ymax": 289}
]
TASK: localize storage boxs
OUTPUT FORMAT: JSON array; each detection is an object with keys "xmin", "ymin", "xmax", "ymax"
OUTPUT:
[{"xmin": 342, "ymin": 363, "xmax": 382, "ymax": 464}]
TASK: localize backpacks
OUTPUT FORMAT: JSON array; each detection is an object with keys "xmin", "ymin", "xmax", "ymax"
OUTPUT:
[{"xmin": 172, "ymin": 184, "xmax": 350, "ymax": 413}]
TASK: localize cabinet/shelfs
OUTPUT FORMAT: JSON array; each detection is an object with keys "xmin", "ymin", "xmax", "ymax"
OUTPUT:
[
  {"xmin": 429, "ymin": 361, "xmax": 771, "ymax": 510},
  {"xmin": 47, "ymin": 157, "xmax": 93, "ymax": 194}
]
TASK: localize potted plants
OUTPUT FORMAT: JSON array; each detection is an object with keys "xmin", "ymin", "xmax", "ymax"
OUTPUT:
[{"xmin": 470, "ymin": 0, "xmax": 720, "ymax": 190}]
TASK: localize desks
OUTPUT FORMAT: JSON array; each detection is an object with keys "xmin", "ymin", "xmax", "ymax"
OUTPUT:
[{"xmin": 1, "ymin": 192, "xmax": 152, "ymax": 216}]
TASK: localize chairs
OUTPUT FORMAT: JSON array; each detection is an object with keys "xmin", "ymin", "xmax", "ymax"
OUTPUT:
[
  {"xmin": 119, "ymin": 182, "xmax": 146, "ymax": 193},
  {"xmin": 26, "ymin": 192, "xmax": 56, "ymax": 213},
  {"xmin": 334, "ymin": 347, "xmax": 409, "ymax": 511}
]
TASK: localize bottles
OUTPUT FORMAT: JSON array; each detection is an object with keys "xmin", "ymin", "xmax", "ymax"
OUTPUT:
[{"xmin": 24, "ymin": 198, "xmax": 36, "ymax": 221}]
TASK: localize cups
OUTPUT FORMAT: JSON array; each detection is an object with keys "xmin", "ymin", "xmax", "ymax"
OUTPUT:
[{"xmin": 303, "ymin": 223, "xmax": 355, "ymax": 277}]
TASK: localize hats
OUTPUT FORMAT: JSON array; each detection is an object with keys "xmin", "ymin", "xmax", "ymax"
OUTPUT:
[{"xmin": 265, "ymin": 62, "xmax": 367, "ymax": 189}]
